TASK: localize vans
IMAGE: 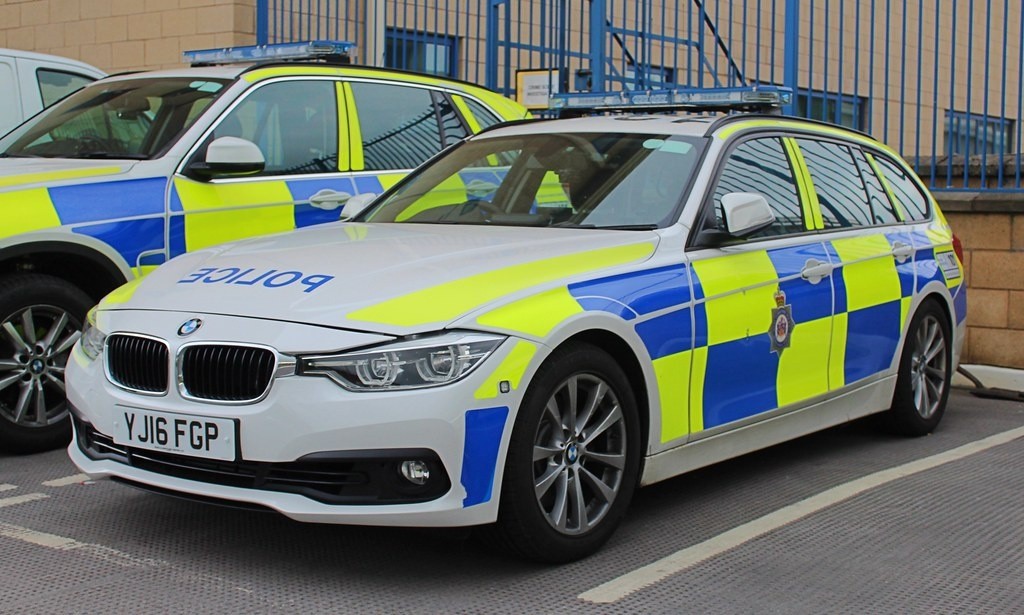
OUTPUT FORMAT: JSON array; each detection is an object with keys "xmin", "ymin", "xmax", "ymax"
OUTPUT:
[{"xmin": 1, "ymin": 47, "xmax": 156, "ymax": 157}]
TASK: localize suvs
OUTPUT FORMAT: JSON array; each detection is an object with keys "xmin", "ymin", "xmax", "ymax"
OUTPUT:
[{"xmin": 0, "ymin": 34, "xmax": 578, "ymax": 460}]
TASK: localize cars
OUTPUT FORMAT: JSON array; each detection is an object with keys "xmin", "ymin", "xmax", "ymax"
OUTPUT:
[{"xmin": 63, "ymin": 82, "xmax": 972, "ymax": 563}]
